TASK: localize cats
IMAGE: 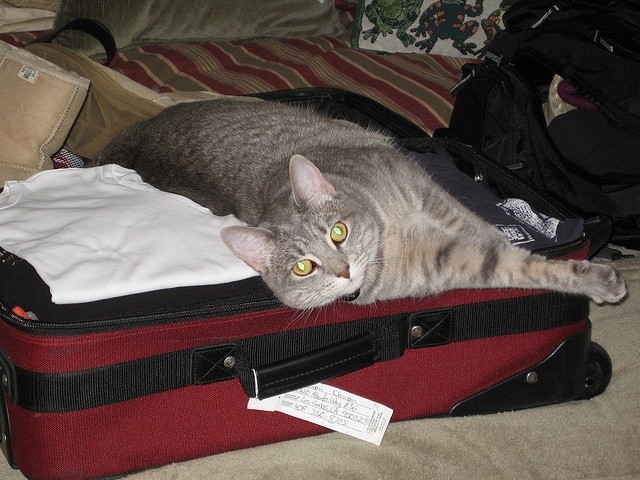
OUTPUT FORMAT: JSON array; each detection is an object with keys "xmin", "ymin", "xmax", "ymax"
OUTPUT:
[{"xmin": 82, "ymin": 93, "xmax": 628, "ymax": 334}]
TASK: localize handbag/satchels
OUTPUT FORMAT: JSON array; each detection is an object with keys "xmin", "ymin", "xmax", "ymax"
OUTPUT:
[{"xmin": 433, "ymin": 0, "xmax": 640, "ymax": 261}]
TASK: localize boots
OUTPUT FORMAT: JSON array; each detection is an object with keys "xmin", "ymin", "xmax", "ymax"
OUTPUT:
[
  {"xmin": 22, "ymin": 43, "xmax": 236, "ymax": 161},
  {"xmin": 1, "ymin": 40, "xmax": 90, "ymax": 190}
]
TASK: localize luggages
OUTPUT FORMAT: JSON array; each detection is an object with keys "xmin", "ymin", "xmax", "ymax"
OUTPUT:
[{"xmin": 0, "ymin": 87, "xmax": 611, "ymax": 480}]
